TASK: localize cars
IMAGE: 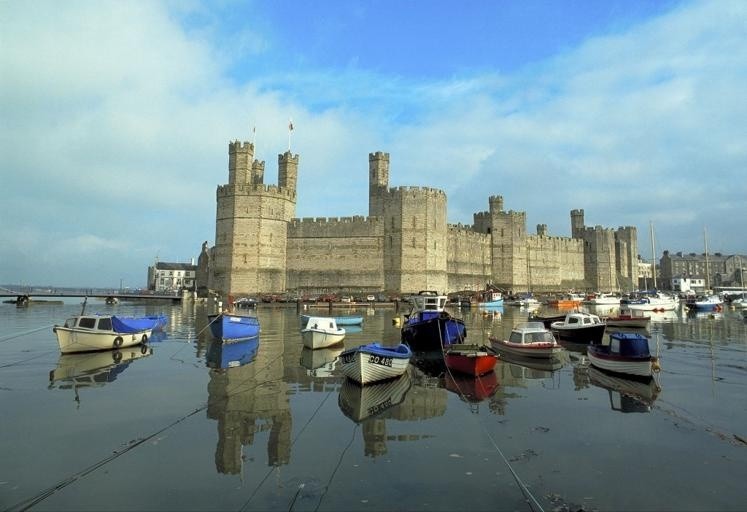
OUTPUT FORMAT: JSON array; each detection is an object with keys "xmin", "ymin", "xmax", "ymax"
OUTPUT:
[
  {"xmin": 341, "ymin": 295, "xmax": 400, "ymax": 302},
  {"xmin": 232, "ymin": 297, "xmax": 258, "ymax": 307},
  {"xmin": 262, "ymin": 294, "xmax": 329, "ymax": 303}
]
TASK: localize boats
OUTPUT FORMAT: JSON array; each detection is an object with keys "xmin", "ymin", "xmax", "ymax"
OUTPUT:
[
  {"xmin": 441, "ymin": 369, "xmax": 501, "ymax": 401},
  {"xmin": 585, "ymin": 363, "xmax": 661, "ymax": 413},
  {"xmin": 401, "ymin": 291, "xmax": 466, "ymax": 352},
  {"xmin": 458, "ymin": 224, "xmax": 747, "ymax": 311},
  {"xmin": 338, "ymin": 373, "xmax": 415, "ymax": 424}
]
